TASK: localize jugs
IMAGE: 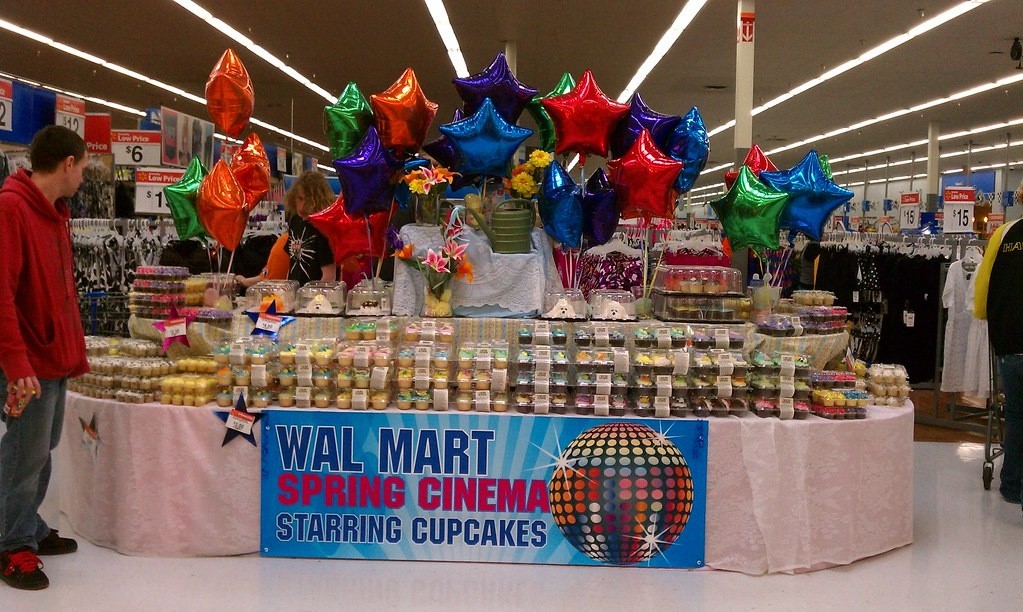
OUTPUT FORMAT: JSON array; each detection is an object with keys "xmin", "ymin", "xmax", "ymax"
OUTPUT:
[{"xmin": 467, "ymin": 198, "xmax": 536, "ymax": 254}]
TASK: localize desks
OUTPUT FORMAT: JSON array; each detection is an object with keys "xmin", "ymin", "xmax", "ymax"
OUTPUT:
[{"xmin": 59, "ymin": 389, "xmax": 914, "ymax": 576}]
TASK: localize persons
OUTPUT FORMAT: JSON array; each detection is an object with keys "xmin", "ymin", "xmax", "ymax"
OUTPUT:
[
  {"xmin": 284, "ymin": 170, "xmax": 336, "ymax": 283},
  {"xmin": 0, "ymin": 125, "xmax": 89, "ymax": 590},
  {"xmin": 234, "ymin": 231, "xmax": 292, "ymax": 288}
]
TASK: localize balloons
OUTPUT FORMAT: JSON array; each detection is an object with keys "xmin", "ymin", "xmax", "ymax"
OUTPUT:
[{"xmin": 163, "ymin": 48, "xmax": 854, "ymax": 297}]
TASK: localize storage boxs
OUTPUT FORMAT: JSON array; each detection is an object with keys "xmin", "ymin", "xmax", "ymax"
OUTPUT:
[
  {"xmin": 127, "ymin": 266, "xmax": 513, "ymax": 413},
  {"xmin": 512, "ymin": 264, "xmax": 911, "ymax": 420}
]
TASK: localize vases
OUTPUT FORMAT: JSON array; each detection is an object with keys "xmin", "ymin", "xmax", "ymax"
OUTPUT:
[
  {"xmin": 419, "ymin": 271, "xmax": 457, "ymax": 318},
  {"xmin": 415, "ymin": 193, "xmax": 441, "ymax": 227}
]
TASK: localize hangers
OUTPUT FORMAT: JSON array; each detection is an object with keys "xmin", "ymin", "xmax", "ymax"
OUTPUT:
[
  {"xmin": 240, "ymin": 200, "xmax": 289, "ymax": 244},
  {"xmin": 66, "ymin": 217, "xmax": 174, "ymax": 244},
  {"xmin": 584, "ymin": 229, "xmax": 724, "ymax": 258},
  {"xmin": 779, "ymin": 231, "xmax": 984, "ymax": 264}
]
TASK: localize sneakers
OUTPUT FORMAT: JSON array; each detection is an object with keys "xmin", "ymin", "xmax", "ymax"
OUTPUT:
[
  {"xmin": 1, "ymin": 547, "xmax": 49, "ymax": 590},
  {"xmin": 36, "ymin": 529, "xmax": 78, "ymax": 555}
]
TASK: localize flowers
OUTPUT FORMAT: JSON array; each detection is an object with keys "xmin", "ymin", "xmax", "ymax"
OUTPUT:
[
  {"xmin": 397, "ymin": 163, "xmax": 463, "ymax": 195},
  {"xmin": 502, "ymin": 148, "xmax": 554, "ymax": 210},
  {"xmin": 382, "ymin": 224, "xmax": 473, "ymax": 284}
]
